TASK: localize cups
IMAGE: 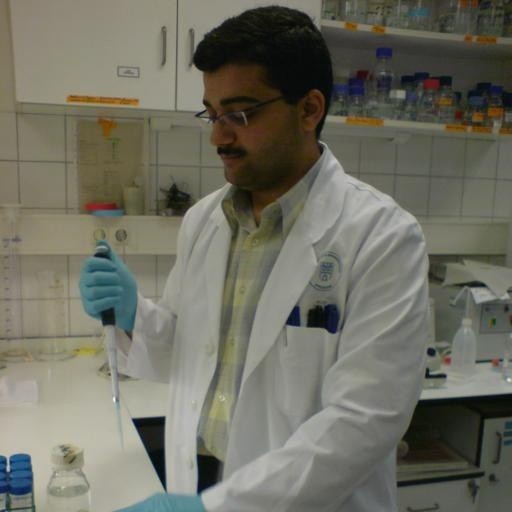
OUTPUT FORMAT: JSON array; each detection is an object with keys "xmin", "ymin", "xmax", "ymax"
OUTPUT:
[{"xmin": 122, "ymin": 186, "xmax": 146, "ymax": 216}]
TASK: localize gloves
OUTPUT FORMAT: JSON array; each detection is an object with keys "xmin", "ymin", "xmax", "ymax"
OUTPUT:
[
  {"xmin": 115, "ymin": 492, "xmax": 206, "ymax": 512},
  {"xmin": 79, "ymin": 240, "xmax": 138, "ymax": 330}
]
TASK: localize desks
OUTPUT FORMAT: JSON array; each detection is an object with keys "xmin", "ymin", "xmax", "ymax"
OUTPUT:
[{"xmin": 0, "ymin": 351, "xmax": 512, "ymax": 512}]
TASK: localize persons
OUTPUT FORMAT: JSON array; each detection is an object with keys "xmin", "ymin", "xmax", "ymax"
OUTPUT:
[{"xmin": 76, "ymin": 3, "xmax": 433, "ymax": 510}]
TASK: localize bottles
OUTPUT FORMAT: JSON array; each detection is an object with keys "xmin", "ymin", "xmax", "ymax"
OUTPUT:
[
  {"xmin": 321, "ymin": 1, "xmax": 510, "ymax": 127},
  {"xmin": 448, "ymin": 319, "xmax": 477, "ymax": 392},
  {"xmin": 0, "ymin": 445, "xmax": 93, "ymax": 511}
]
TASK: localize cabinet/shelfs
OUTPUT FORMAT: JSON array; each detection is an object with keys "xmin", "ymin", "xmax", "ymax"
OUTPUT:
[
  {"xmin": 10, "ymin": 0, "xmax": 315, "ymax": 118},
  {"xmin": 316, "ymin": 0, "xmax": 512, "ymax": 142}
]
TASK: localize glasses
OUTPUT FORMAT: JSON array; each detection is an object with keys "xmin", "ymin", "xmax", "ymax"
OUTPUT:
[{"xmin": 194, "ymin": 96, "xmax": 284, "ymax": 131}]
